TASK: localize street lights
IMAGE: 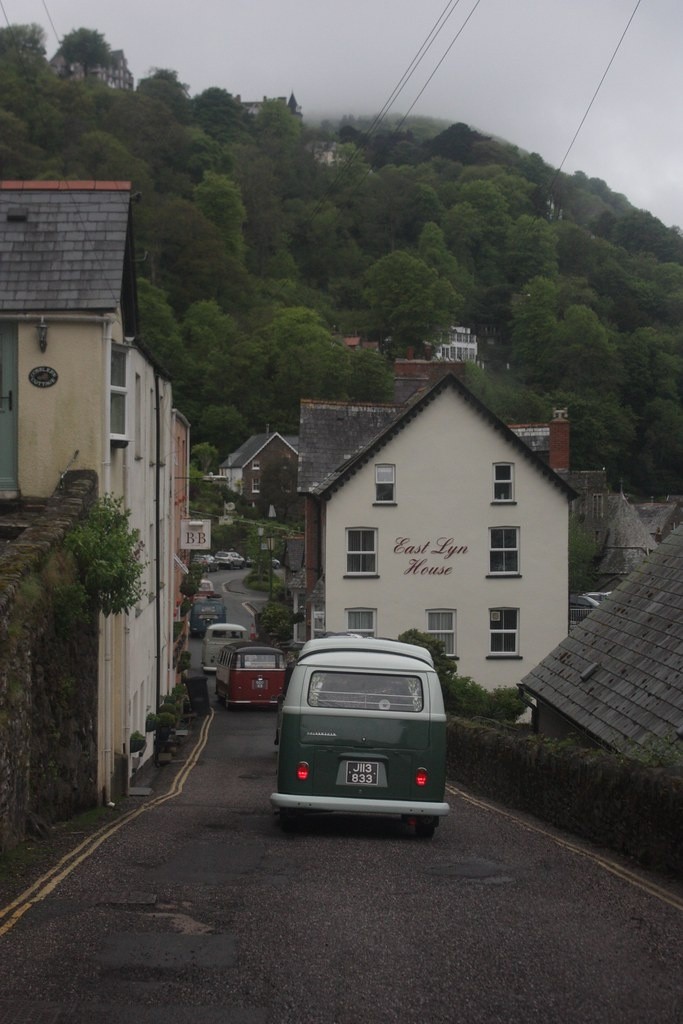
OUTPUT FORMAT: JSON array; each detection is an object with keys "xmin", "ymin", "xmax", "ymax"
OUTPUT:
[
  {"xmin": 257, "ymin": 525, "xmax": 265, "ymax": 562},
  {"xmin": 266, "ymin": 533, "xmax": 275, "ymax": 601}
]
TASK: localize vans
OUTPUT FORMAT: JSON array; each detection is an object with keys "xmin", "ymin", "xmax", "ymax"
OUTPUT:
[
  {"xmin": 272, "ymin": 634, "xmax": 451, "ymax": 838},
  {"xmin": 273, "ymin": 640, "xmax": 306, "ymax": 689},
  {"xmin": 200, "ymin": 623, "xmax": 248, "ymax": 676},
  {"xmin": 188, "ymin": 593, "xmax": 228, "ymax": 639},
  {"xmin": 188, "ymin": 579, "xmax": 213, "ymax": 607},
  {"xmin": 214, "ymin": 642, "xmax": 287, "ymax": 713}
]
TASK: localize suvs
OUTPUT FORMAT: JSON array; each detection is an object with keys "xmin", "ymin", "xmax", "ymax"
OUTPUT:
[{"xmin": 215, "ymin": 551, "xmax": 246, "ymax": 571}]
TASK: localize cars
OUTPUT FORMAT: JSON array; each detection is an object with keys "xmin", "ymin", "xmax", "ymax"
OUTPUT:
[
  {"xmin": 191, "ymin": 554, "xmax": 220, "ymax": 574},
  {"xmin": 247, "ymin": 554, "xmax": 281, "ymax": 569}
]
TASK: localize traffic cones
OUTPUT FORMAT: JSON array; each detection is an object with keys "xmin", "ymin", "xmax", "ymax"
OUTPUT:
[{"xmin": 250, "ymin": 623, "xmax": 257, "ymax": 641}]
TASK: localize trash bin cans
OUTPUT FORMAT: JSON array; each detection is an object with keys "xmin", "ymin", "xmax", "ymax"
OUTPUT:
[{"xmin": 185, "ymin": 676, "xmax": 211, "ymax": 716}]
